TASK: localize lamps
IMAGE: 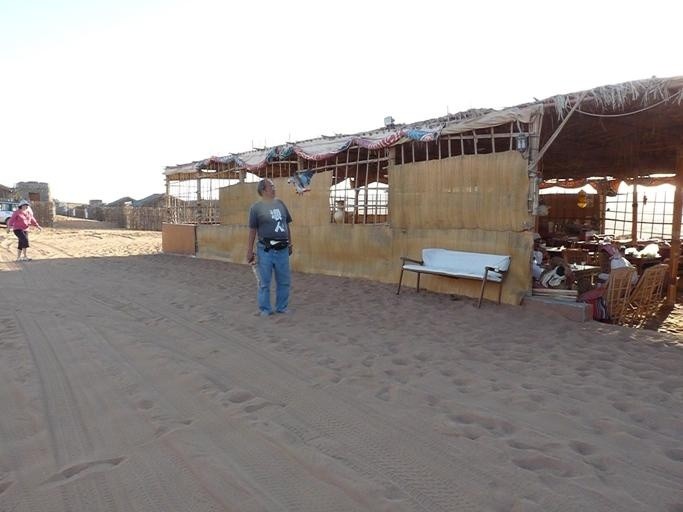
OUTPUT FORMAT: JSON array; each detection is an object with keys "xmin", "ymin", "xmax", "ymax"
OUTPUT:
[
  {"xmin": 515, "ymin": 132, "xmax": 532, "ymax": 165},
  {"xmin": 577, "ymin": 189, "xmax": 589, "ymax": 209}
]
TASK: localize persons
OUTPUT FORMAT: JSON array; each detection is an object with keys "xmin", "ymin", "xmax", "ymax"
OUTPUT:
[
  {"xmin": 6, "ymin": 199, "xmax": 44, "ymax": 263},
  {"xmin": 575, "ymin": 242, "xmax": 639, "ymax": 322},
  {"xmin": 245, "ymin": 178, "xmax": 294, "ymax": 317}
]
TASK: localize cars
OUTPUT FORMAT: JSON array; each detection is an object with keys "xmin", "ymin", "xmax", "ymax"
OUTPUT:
[{"xmin": 0, "ymin": 202, "xmax": 33, "ymax": 227}]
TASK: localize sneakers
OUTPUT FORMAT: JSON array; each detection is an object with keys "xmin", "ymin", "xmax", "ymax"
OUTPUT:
[
  {"xmin": 258, "ymin": 310, "xmax": 292, "ymax": 317},
  {"xmin": 15, "ymin": 256, "xmax": 32, "ymax": 263}
]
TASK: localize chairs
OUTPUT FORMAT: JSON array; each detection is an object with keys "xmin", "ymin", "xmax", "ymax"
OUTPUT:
[{"xmin": 533, "ymin": 235, "xmax": 683, "ymax": 328}]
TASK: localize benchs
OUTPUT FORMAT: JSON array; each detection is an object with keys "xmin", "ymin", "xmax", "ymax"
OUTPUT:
[{"xmin": 394, "ymin": 247, "xmax": 511, "ymax": 311}]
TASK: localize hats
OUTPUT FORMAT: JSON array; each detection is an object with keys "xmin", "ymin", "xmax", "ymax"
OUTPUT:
[{"xmin": 17, "ymin": 200, "xmax": 30, "ymax": 208}]
